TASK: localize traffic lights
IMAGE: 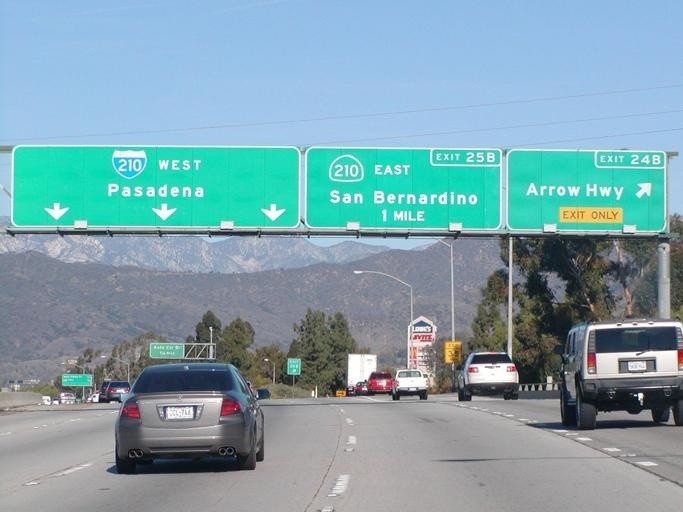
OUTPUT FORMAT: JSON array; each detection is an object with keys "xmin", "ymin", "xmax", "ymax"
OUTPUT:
[{"xmin": 148, "ymin": 342, "xmax": 186, "ymax": 361}]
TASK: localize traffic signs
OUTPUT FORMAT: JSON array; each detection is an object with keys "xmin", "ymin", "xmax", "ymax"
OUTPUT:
[
  {"xmin": 286, "ymin": 357, "xmax": 301, "ymax": 376},
  {"xmin": 60, "ymin": 373, "xmax": 93, "ymax": 387},
  {"xmin": 8, "ymin": 143, "xmax": 300, "ymax": 230},
  {"xmin": 303, "ymin": 146, "xmax": 504, "ymax": 233},
  {"xmin": 504, "ymin": 149, "xmax": 669, "ymax": 237},
  {"xmin": 443, "ymin": 342, "xmax": 462, "ymax": 364}
]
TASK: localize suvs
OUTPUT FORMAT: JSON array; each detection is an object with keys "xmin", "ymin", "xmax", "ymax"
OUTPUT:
[
  {"xmin": 452, "ymin": 348, "xmax": 521, "ymax": 404},
  {"xmin": 557, "ymin": 316, "xmax": 682, "ymax": 433},
  {"xmin": 344, "ymin": 367, "xmax": 430, "ymax": 403}
]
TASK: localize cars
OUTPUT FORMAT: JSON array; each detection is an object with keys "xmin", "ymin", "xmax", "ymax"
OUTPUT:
[
  {"xmin": 112, "ymin": 357, "xmax": 273, "ymax": 474},
  {"xmin": 38, "ymin": 381, "xmax": 135, "ymax": 406}
]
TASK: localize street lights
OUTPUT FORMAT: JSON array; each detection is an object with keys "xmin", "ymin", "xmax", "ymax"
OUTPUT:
[
  {"xmin": 351, "ymin": 269, "xmax": 413, "ymax": 371},
  {"xmin": 207, "ymin": 325, "xmax": 214, "ymax": 363},
  {"xmin": 262, "ymin": 358, "xmax": 274, "ymax": 385},
  {"xmin": 99, "ymin": 355, "xmax": 129, "ymax": 382},
  {"xmin": 60, "ymin": 362, "xmax": 85, "ymax": 404}
]
